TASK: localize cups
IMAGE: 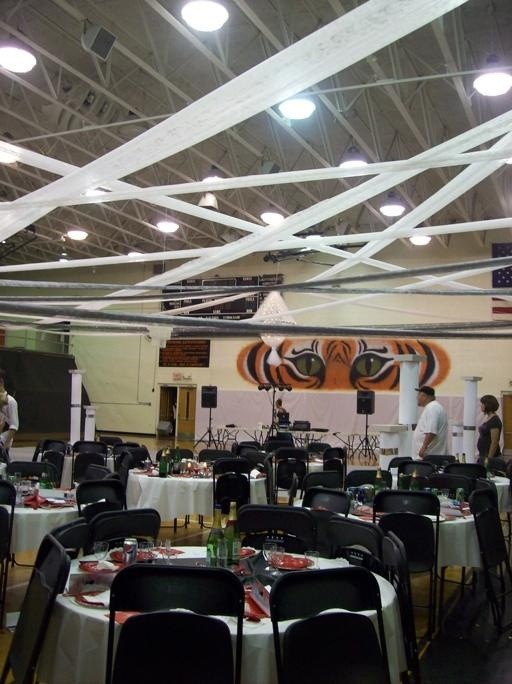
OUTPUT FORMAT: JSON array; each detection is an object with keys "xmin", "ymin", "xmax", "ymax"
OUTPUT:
[{"xmin": 302, "ymin": 552, "xmax": 319, "ymax": 571}]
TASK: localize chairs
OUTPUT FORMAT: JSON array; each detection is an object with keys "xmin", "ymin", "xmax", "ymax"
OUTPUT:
[{"xmin": 2, "ymin": 424, "xmax": 511, "ymax": 683}]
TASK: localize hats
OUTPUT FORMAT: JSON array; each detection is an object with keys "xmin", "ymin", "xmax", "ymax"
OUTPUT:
[{"xmin": 414, "ymin": 386, "xmax": 435, "ymax": 396}]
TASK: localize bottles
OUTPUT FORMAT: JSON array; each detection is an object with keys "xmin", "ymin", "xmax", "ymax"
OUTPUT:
[
  {"xmin": 483, "ymin": 457, "xmax": 490, "ymax": 477},
  {"xmin": 373, "ymin": 466, "xmax": 382, "ymax": 495},
  {"xmin": 396, "ymin": 472, "xmax": 406, "ymax": 491},
  {"xmin": 409, "ymin": 468, "xmax": 420, "ymax": 493},
  {"xmin": 226, "ymin": 502, "xmax": 240, "ymax": 567},
  {"xmin": 455, "ymin": 452, "xmax": 466, "ymax": 464},
  {"xmin": 40, "ymin": 460, "xmax": 52, "ymax": 490},
  {"xmin": 158, "ymin": 446, "xmax": 182, "ymax": 477},
  {"xmin": 205, "ymin": 504, "xmax": 226, "ymax": 569}
]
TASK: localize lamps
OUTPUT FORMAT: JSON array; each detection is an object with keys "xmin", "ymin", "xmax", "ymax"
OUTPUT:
[{"xmin": 1, "ymin": 0, "xmax": 511, "ymax": 267}]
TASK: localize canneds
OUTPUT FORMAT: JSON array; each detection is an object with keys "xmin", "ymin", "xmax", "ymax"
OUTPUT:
[
  {"xmin": 456, "ymin": 488, "xmax": 465, "ymax": 502},
  {"xmin": 123, "ymin": 538, "xmax": 139, "ymax": 565}
]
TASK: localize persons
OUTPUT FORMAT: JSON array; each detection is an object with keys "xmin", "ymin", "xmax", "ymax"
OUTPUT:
[
  {"xmin": 1, "ymin": 371, "xmax": 22, "ymax": 465},
  {"xmin": 475, "ymin": 393, "xmax": 504, "ymax": 466},
  {"xmin": 412, "ymin": 386, "xmax": 451, "ymax": 458},
  {"xmin": 273, "ymin": 397, "xmax": 286, "ymax": 424}
]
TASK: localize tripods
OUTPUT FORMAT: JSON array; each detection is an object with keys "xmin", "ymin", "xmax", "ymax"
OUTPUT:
[
  {"xmin": 265, "ymin": 387, "xmax": 278, "ymax": 443},
  {"xmin": 193, "ymin": 408, "xmax": 219, "ymax": 450},
  {"xmin": 351, "ymin": 414, "xmax": 378, "ymax": 461}
]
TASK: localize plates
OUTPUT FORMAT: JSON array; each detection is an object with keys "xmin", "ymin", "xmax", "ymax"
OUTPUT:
[
  {"xmin": 76, "ymin": 590, "xmax": 112, "ymax": 607},
  {"xmin": 274, "ymin": 553, "xmax": 313, "ymax": 571},
  {"xmin": 160, "ymin": 547, "xmax": 184, "ymax": 556},
  {"xmin": 111, "ymin": 549, "xmax": 155, "ymax": 563},
  {"xmin": 78, "ymin": 562, "xmax": 126, "ymax": 574},
  {"xmin": 241, "ymin": 548, "xmax": 256, "ymax": 559}
]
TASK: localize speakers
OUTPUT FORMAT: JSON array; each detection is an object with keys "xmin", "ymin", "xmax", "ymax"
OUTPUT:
[
  {"xmin": 357, "ymin": 391, "xmax": 375, "ymax": 414},
  {"xmin": 201, "ymin": 385, "xmax": 217, "ymax": 408}
]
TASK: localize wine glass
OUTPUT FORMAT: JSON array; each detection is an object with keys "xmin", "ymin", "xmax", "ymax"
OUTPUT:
[
  {"xmin": 338, "ymin": 483, "xmax": 454, "ymax": 521},
  {"xmin": 94, "ymin": 539, "xmax": 108, "ymax": 571},
  {"xmin": 182, "ymin": 457, "xmax": 212, "ymax": 479},
  {"xmin": 5, "ymin": 470, "xmax": 39, "ymax": 511},
  {"xmin": 263, "ymin": 542, "xmax": 284, "ymax": 574},
  {"xmin": 138, "ymin": 538, "xmax": 173, "ymax": 566}
]
